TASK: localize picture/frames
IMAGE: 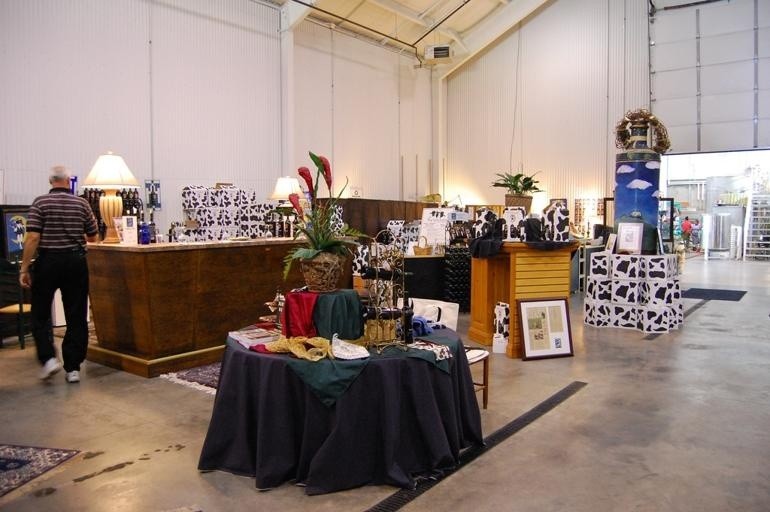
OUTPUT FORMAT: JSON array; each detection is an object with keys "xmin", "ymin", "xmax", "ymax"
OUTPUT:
[
  {"xmin": 604, "ymin": 222, "xmax": 644, "ymax": 255},
  {"xmin": 0, "ymin": 205, "xmax": 31, "ymax": 263},
  {"xmin": 516, "ymin": 296, "xmax": 575, "ymax": 361}
]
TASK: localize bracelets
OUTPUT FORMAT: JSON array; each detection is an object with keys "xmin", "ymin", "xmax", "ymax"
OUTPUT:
[{"xmin": 19, "ymin": 269, "xmax": 31, "ymax": 273}]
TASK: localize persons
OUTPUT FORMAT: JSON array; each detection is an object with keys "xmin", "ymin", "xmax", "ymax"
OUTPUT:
[
  {"xmin": 17, "ymin": 164, "xmax": 99, "ymax": 384},
  {"xmin": 681, "ymin": 216, "xmax": 692, "ymax": 249},
  {"xmin": 690, "ymin": 219, "xmax": 702, "ymax": 246}
]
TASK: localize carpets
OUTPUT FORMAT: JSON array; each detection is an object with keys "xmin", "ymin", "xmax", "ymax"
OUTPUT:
[
  {"xmin": 160, "ymin": 360, "xmax": 222, "ymax": 396},
  {"xmin": 0, "ymin": 441, "xmax": 81, "ymax": 496}
]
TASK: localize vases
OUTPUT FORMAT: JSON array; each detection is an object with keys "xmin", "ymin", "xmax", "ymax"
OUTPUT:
[{"xmin": 295, "ymin": 252, "xmax": 347, "ymax": 294}]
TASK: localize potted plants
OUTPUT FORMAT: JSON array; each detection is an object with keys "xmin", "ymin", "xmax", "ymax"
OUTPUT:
[{"xmin": 492, "ymin": 170, "xmax": 546, "ymax": 216}]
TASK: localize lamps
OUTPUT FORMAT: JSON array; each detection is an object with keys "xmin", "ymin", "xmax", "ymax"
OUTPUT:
[
  {"xmin": 81, "ymin": 151, "xmax": 141, "ymax": 244},
  {"xmin": 269, "ymin": 177, "xmax": 307, "ymax": 203}
]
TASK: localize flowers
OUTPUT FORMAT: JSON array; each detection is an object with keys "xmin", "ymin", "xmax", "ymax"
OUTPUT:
[{"xmin": 268, "ymin": 150, "xmax": 375, "ymax": 282}]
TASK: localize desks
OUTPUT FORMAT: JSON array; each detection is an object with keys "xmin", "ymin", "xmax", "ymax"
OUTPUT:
[{"xmin": 225, "ymin": 322, "xmax": 462, "ymax": 484}]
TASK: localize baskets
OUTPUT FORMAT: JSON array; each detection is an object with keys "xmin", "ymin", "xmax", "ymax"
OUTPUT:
[{"xmin": 413, "ymin": 235, "xmax": 433, "ymax": 256}]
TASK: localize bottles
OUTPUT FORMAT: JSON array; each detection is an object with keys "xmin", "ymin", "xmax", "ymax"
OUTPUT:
[
  {"xmin": 83, "ymin": 187, "xmax": 143, "ymax": 218},
  {"xmin": 137, "ymin": 209, "xmax": 156, "ymax": 245},
  {"xmin": 149, "ymin": 185, "xmax": 158, "ymax": 208}
]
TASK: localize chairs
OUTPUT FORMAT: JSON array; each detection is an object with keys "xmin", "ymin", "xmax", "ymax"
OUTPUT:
[{"xmin": 0, "ymin": 255, "xmax": 32, "ymax": 350}]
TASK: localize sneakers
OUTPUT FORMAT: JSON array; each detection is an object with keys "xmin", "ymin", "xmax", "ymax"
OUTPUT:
[
  {"xmin": 39, "ymin": 357, "xmax": 61, "ymax": 380},
  {"xmin": 64, "ymin": 370, "xmax": 81, "ymax": 383}
]
